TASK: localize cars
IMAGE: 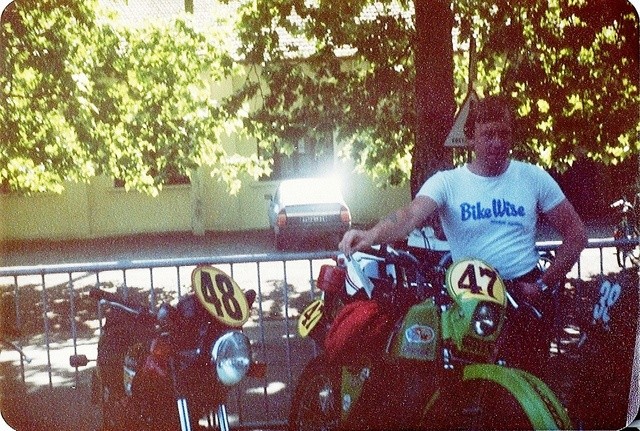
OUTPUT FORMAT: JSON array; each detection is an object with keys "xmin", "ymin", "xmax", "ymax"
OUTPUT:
[{"xmin": 264, "ymin": 178, "xmax": 351, "ymax": 251}]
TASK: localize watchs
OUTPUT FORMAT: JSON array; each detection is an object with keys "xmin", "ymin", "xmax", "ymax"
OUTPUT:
[{"xmin": 535, "ymin": 278, "xmax": 549, "ymax": 297}]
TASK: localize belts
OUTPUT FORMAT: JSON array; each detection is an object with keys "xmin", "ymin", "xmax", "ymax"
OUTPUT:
[{"xmin": 500, "ymin": 268, "xmax": 541, "ymax": 287}]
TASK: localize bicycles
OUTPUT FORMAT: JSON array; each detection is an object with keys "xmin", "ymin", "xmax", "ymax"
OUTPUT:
[{"xmin": 610, "ymin": 195, "xmax": 640, "ymax": 267}]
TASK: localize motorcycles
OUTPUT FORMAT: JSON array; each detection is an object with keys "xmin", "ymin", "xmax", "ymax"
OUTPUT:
[
  {"xmin": 70, "ymin": 266, "xmax": 266, "ymax": 431},
  {"xmin": 290, "ymin": 240, "xmax": 572, "ymax": 430}
]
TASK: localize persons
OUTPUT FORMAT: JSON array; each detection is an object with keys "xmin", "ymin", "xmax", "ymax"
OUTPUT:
[{"xmin": 339, "ymin": 99, "xmax": 587, "ymax": 301}]
